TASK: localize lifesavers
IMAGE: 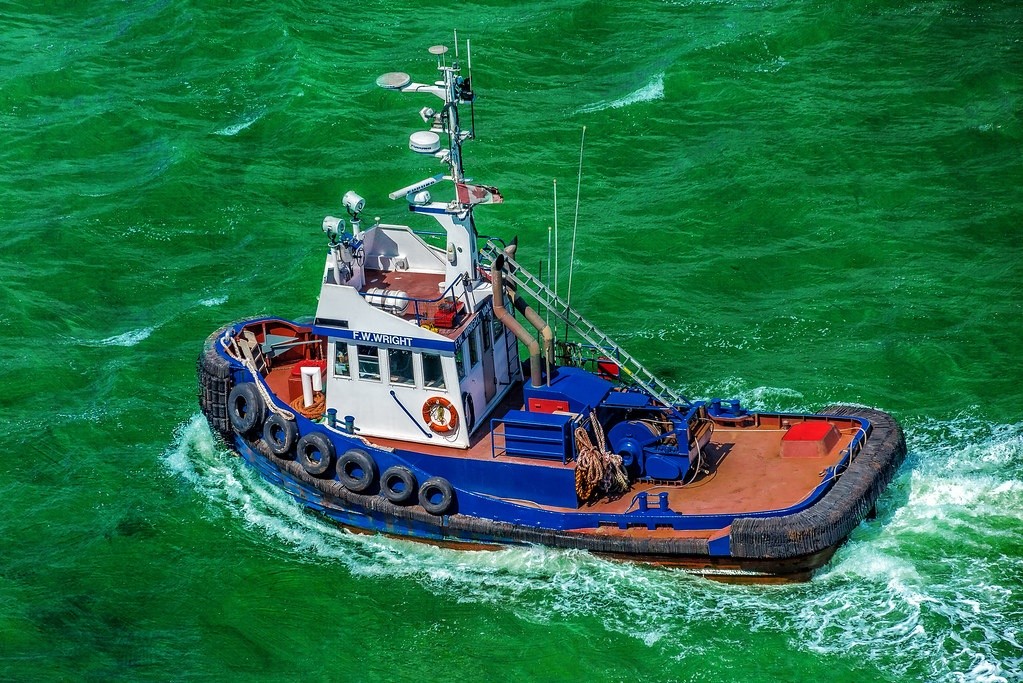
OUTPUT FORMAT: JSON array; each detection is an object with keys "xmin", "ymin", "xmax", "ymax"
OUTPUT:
[
  {"xmin": 336, "ymin": 448, "xmax": 379, "ymax": 493},
  {"xmin": 419, "ymin": 477, "xmax": 456, "ymax": 516},
  {"xmin": 422, "ymin": 396, "xmax": 458, "ymax": 434},
  {"xmin": 263, "ymin": 413, "xmax": 300, "ymax": 458},
  {"xmin": 379, "ymin": 466, "xmax": 419, "ymax": 504},
  {"xmin": 296, "ymin": 432, "xmax": 336, "ymax": 476},
  {"xmin": 227, "ymin": 381, "xmax": 269, "ymax": 435}
]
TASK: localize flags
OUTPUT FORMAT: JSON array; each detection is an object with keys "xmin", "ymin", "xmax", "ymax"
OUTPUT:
[{"xmin": 455, "ymin": 183, "xmax": 503, "ymax": 204}]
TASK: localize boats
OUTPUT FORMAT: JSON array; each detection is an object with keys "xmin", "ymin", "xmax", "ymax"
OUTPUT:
[{"xmin": 198, "ymin": 28, "xmax": 907, "ymax": 584}]
